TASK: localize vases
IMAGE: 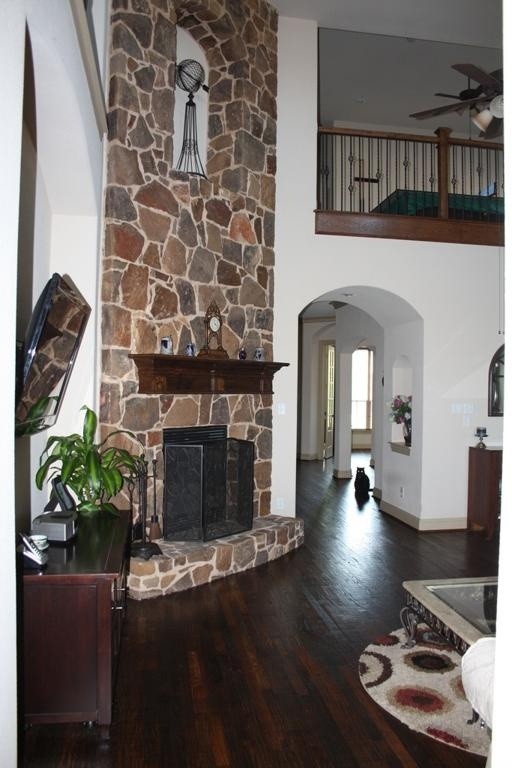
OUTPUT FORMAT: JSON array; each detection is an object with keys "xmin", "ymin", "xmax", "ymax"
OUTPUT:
[{"xmin": 402, "ymin": 423, "xmax": 411, "ymax": 446}]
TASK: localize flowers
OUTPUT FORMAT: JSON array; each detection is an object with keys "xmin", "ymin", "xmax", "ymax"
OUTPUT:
[{"xmin": 385, "ymin": 394, "xmax": 412, "ymax": 434}]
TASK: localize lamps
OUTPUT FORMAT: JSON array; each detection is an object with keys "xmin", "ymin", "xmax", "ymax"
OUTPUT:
[{"xmin": 471, "ymin": 108, "xmax": 494, "ymax": 132}]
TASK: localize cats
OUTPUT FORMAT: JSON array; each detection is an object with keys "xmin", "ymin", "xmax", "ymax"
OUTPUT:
[{"xmin": 354, "ymin": 467, "xmax": 374, "ymax": 495}]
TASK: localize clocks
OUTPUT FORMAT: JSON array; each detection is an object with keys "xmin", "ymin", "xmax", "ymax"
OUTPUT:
[{"xmin": 198, "ymin": 298, "xmax": 229, "ymax": 360}]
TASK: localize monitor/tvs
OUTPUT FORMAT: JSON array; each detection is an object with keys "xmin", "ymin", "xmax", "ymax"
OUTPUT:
[{"xmin": 15, "ymin": 273, "xmax": 91, "ymax": 439}]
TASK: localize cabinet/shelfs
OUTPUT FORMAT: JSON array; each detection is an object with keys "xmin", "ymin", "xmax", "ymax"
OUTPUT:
[
  {"xmin": 18, "ymin": 509, "xmax": 134, "ymax": 726},
  {"xmin": 464, "ymin": 445, "xmax": 503, "ymax": 541}
]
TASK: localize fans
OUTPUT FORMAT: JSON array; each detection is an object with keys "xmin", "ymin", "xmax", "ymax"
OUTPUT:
[{"xmin": 406, "ymin": 59, "xmax": 504, "ymax": 137}]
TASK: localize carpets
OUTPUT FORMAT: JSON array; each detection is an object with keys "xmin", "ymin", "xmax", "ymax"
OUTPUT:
[{"xmin": 357, "ymin": 618, "xmax": 496, "ymax": 758}]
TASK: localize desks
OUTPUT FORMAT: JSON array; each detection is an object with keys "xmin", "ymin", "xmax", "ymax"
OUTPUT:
[{"xmin": 369, "ymin": 188, "xmax": 504, "ymax": 224}]
